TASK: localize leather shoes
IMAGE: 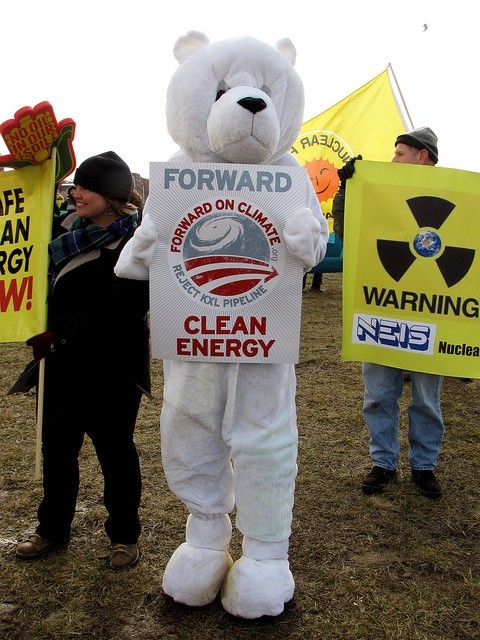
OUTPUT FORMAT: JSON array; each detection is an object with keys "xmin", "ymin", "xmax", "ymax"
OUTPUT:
[
  {"xmin": 15, "ymin": 532, "xmax": 52, "ymax": 558},
  {"xmin": 110, "ymin": 544, "xmax": 139, "ymax": 569}
]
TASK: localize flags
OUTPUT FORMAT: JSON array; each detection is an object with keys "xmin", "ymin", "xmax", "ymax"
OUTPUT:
[
  {"xmin": 286, "ymin": 68, "xmax": 409, "ymax": 236},
  {"xmin": 0, "ymin": 156, "xmax": 58, "ymax": 344},
  {"xmin": 340, "ymin": 155, "xmax": 479, "ymax": 380}
]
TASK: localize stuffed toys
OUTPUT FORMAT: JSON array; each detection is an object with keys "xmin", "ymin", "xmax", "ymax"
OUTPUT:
[{"xmin": 115, "ymin": 29, "xmax": 328, "ymax": 623}]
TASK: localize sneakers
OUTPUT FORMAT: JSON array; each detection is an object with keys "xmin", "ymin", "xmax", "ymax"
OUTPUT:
[
  {"xmin": 412, "ymin": 469, "xmax": 442, "ymax": 497},
  {"xmin": 360, "ymin": 466, "xmax": 397, "ymax": 492}
]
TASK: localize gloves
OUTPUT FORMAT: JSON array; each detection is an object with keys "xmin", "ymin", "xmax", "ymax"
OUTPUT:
[
  {"xmin": 337, "ymin": 154, "xmax": 362, "ymax": 189},
  {"xmin": 26, "ymin": 328, "xmax": 73, "ymax": 361}
]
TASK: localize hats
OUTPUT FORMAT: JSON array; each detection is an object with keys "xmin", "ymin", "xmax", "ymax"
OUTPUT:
[
  {"xmin": 73, "ymin": 151, "xmax": 132, "ymax": 203},
  {"xmin": 394, "ymin": 126, "xmax": 439, "ymax": 164}
]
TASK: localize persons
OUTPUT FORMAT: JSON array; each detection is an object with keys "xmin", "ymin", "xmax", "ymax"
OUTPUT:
[
  {"xmin": 60, "ymin": 185, "xmax": 78, "ymax": 216},
  {"xmin": 13, "ymin": 152, "xmax": 153, "ymax": 571},
  {"xmin": 302, "ymin": 271, "xmax": 326, "ymax": 295},
  {"xmin": 332, "ymin": 125, "xmax": 447, "ymax": 499}
]
